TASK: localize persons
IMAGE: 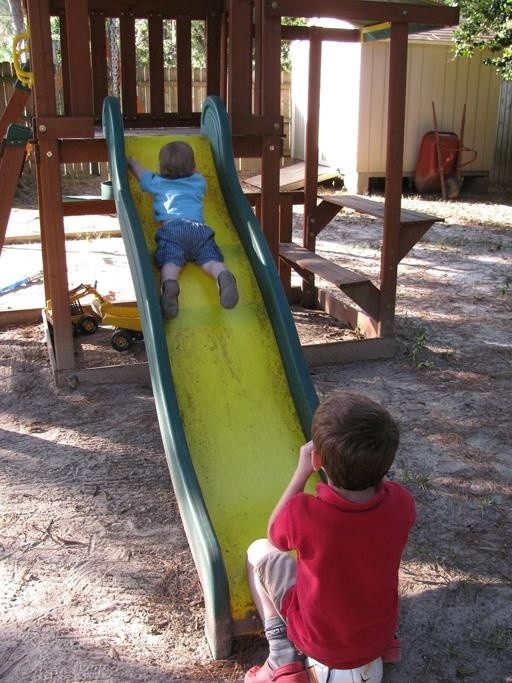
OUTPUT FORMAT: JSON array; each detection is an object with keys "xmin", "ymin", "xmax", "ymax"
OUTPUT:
[
  {"xmin": 125, "ymin": 141, "xmax": 239, "ymax": 319},
  {"xmin": 243, "ymin": 387, "xmax": 419, "ymax": 681}
]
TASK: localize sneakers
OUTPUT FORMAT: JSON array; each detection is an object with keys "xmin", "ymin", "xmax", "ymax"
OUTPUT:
[
  {"xmin": 217, "ymin": 271, "xmax": 238, "ymax": 309},
  {"xmin": 244, "ymin": 658, "xmax": 309, "ymax": 683},
  {"xmin": 160, "ymin": 279, "xmax": 179, "ymax": 319},
  {"xmin": 383, "ymin": 633, "xmax": 401, "ymax": 664}
]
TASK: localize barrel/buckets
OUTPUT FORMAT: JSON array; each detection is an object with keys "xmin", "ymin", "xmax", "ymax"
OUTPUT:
[{"xmin": 461, "ymin": 170, "xmax": 490, "ymax": 200}]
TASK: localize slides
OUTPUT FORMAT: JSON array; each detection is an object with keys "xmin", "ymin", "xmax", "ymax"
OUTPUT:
[{"xmin": 102, "ymin": 96, "xmax": 327, "ymax": 661}]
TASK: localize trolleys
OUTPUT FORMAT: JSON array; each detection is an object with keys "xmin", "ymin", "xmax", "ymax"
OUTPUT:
[{"xmin": 415, "ymin": 100, "xmax": 477, "ymax": 201}]
